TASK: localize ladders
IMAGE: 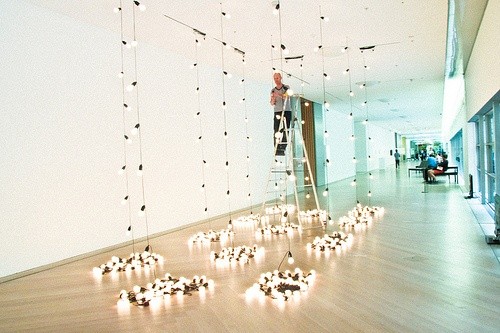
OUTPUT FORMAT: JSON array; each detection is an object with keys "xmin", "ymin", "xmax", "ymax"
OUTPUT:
[{"xmin": 259, "ymin": 94, "xmax": 326, "ymax": 238}]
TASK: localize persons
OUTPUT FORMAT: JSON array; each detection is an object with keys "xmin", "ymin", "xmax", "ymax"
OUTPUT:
[
  {"xmin": 270, "ymin": 72, "xmax": 292, "ymax": 155},
  {"xmin": 426, "ymin": 154, "xmax": 448, "ymax": 182},
  {"xmin": 414, "ymin": 147, "xmax": 446, "ymax": 160},
  {"xmin": 425, "ymin": 154, "xmax": 436, "ymax": 182},
  {"xmin": 438, "ymin": 154, "xmax": 444, "ymax": 164},
  {"xmin": 394, "ymin": 149, "xmax": 401, "ymax": 168}
]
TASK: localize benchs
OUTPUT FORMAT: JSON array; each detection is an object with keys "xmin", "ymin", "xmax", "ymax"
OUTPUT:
[
  {"xmin": 409, "ymin": 168, "xmax": 425, "ymax": 179},
  {"xmin": 430, "ymin": 172, "xmax": 459, "ymax": 184},
  {"xmin": 448, "ymin": 166, "xmax": 459, "ymax": 175},
  {"xmin": 416, "ymin": 165, "xmax": 423, "ymax": 174}
]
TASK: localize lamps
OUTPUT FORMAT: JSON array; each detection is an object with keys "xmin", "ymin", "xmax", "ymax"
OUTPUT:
[{"xmin": 92, "ymin": 1, "xmax": 384, "ymax": 315}]
